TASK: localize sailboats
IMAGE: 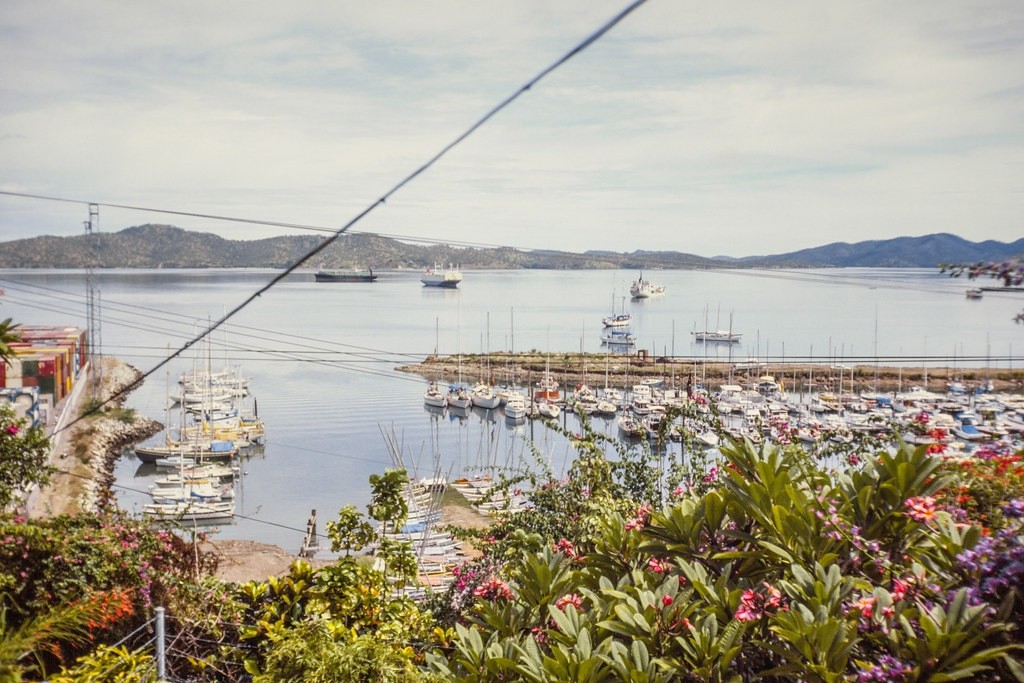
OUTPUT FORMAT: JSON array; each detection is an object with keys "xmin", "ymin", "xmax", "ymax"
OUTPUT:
[{"xmin": 422, "ymin": 307, "xmax": 1024, "ymax": 450}]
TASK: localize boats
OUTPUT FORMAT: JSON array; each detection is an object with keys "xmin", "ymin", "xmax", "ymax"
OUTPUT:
[
  {"xmin": 600, "ymin": 326, "xmax": 636, "ymax": 346},
  {"xmin": 314, "ymin": 265, "xmax": 380, "ymax": 283},
  {"xmin": 965, "ymin": 286, "xmax": 984, "ymax": 299},
  {"xmin": 689, "ymin": 328, "xmax": 742, "ymax": 343},
  {"xmin": 421, "ymin": 259, "xmax": 463, "ymax": 286},
  {"xmin": 602, "ymin": 296, "xmax": 634, "ymax": 328},
  {"xmin": 130, "ymin": 303, "xmax": 269, "ymax": 538},
  {"xmin": 629, "ymin": 269, "xmax": 656, "ymax": 299}
]
